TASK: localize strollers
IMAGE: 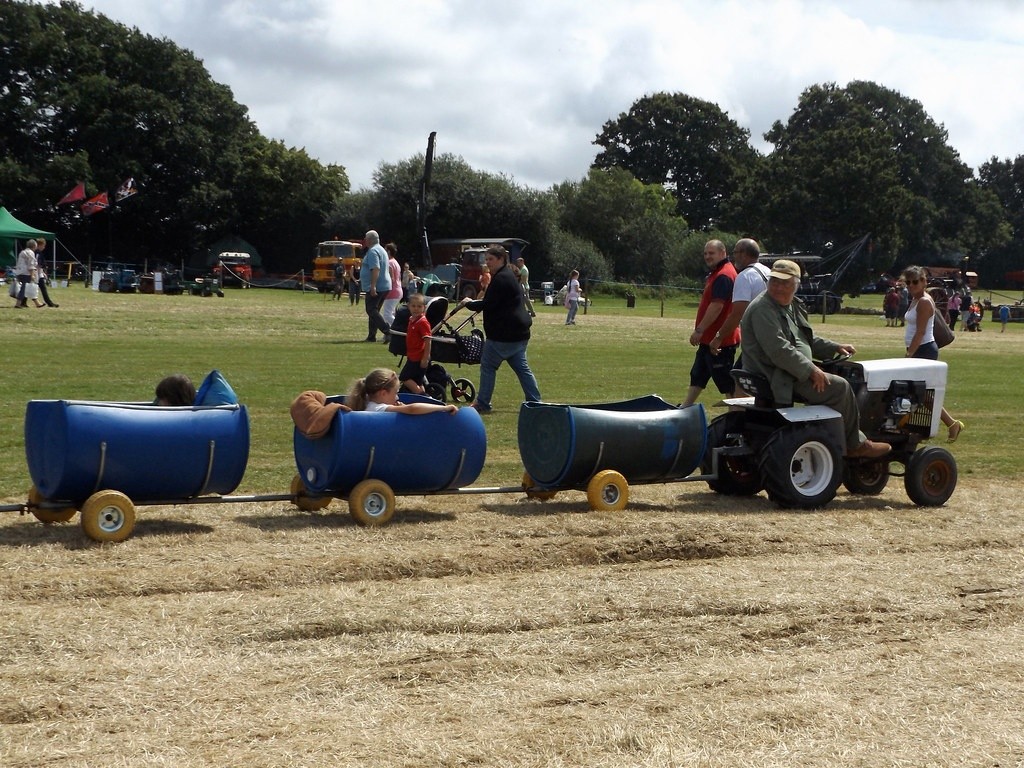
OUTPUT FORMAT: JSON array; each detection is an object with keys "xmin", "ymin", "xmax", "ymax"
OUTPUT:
[{"xmin": 388, "ymin": 298, "xmax": 484, "ymax": 405}]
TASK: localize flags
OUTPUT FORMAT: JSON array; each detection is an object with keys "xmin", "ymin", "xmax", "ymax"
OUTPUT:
[
  {"xmin": 80, "ymin": 192, "xmax": 109, "ymax": 215},
  {"xmin": 58, "ymin": 180, "xmax": 85, "ymax": 205},
  {"xmin": 115, "ymin": 177, "xmax": 138, "ymax": 202}
]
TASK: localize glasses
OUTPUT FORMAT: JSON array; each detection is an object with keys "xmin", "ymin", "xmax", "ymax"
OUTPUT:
[{"xmin": 904, "ymin": 279, "xmax": 922, "ymax": 285}]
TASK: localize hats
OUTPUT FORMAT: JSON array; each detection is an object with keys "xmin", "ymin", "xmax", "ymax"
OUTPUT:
[{"xmin": 766, "ymin": 259, "xmax": 801, "ymax": 282}]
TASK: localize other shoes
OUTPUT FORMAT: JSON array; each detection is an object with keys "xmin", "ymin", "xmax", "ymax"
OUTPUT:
[
  {"xmin": 384, "ymin": 333, "xmax": 391, "ymax": 344},
  {"xmin": 566, "ymin": 319, "xmax": 576, "ymax": 326},
  {"xmin": 472, "ymin": 401, "xmax": 490, "ymax": 412},
  {"xmin": 22, "ymin": 304, "xmax": 29, "ymax": 308},
  {"xmin": 38, "ymin": 303, "xmax": 46, "ymax": 308},
  {"xmin": 363, "ymin": 337, "xmax": 377, "ymax": 343},
  {"xmin": 845, "ymin": 439, "xmax": 891, "ymax": 458},
  {"xmin": 14, "ymin": 303, "xmax": 20, "ymax": 308},
  {"xmin": 50, "ymin": 304, "xmax": 60, "ymax": 308}
]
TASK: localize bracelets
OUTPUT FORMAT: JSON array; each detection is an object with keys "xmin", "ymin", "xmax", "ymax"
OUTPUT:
[
  {"xmin": 696, "ymin": 328, "xmax": 704, "ymax": 333},
  {"xmin": 907, "ymin": 352, "xmax": 912, "ymax": 356}
]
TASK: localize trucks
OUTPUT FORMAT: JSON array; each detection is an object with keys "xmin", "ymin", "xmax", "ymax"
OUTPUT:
[{"xmin": 313, "ymin": 236, "xmax": 364, "ymax": 294}]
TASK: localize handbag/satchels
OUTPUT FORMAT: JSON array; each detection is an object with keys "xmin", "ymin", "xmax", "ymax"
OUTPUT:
[
  {"xmin": 9, "ymin": 280, "xmax": 22, "ymax": 298},
  {"xmin": 454, "ymin": 333, "xmax": 484, "ymax": 365},
  {"xmin": 933, "ymin": 304, "xmax": 955, "ymax": 348},
  {"xmin": 25, "ymin": 279, "xmax": 39, "ymax": 299}
]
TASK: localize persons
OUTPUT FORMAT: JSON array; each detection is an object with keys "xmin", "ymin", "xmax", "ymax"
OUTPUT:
[
  {"xmin": 903, "ymin": 267, "xmax": 965, "ymax": 443},
  {"xmin": 947, "ymin": 292, "xmax": 981, "ymax": 332},
  {"xmin": 565, "ymin": 270, "xmax": 582, "ymax": 325},
  {"xmin": 741, "ymin": 259, "xmax": 893, "ymax": 457},
  {"xmin": 678, "ymin": 239, "xmax": 741, "ymax": 406},
  {"xmin": 885, "ymin": 282, "xmax": 908, "ymax": 327},
  {"xmin": 360, "ymin": 230, "xmax": 403, "ymax": 345},
  {"xmin": 398, "ymin": 293, "xmax": 432, "ymax": 396},
  {"xmin": 477, "ymin": 258, "xmax": 536, "ymax": 317},
  {"xmin": 14, "ymin": 238, "xmax": 59, "ymax": 308},
  {"xmin": 346, "ymin": 367, "xmax": 459, "ymax": 415},
  {"xmin": 399, "ymin": 258, "xmax": 414, "ymax": 303},
  {"xmin": 155, "ymin": 374, "xmax": 196, "ymax": 406},
  {"xmin": 709, "ymin": 237, "xmax": 771, "ymax": 398},
  {"xmin": 349, "ymin": 259, "xmax": 362, "ymax": 306},
  {"xmin": 999, "ymin": 304, "xmax": 1011, "ymax": 333},
  {"xmin": 331, "ymin": 257, "xmax": 347, "ymax": 300},
  {"xmin": 460, "ymin": 245, "xmax": 541, "ymax": 414}
]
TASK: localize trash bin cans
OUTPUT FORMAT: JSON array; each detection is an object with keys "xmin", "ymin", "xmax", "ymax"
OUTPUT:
[{"xmin": 541, "ymin": 282, "xmax": 554, "ymax": 299}]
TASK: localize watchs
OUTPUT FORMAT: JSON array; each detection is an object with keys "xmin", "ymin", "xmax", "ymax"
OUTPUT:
[{"xmin": 716, "ymin": 331, "xmax": 722, "ymax": 339}]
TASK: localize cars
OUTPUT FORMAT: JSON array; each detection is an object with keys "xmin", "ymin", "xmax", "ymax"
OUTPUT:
[{"xmin": 861, "ymin": 283, "xmax": 878, "ymax": 294}]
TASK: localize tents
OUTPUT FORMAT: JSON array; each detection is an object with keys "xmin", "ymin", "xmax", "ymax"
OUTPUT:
[{"xmin": 0, "ymin": 206, "xmax": 56, "ymax": 280}]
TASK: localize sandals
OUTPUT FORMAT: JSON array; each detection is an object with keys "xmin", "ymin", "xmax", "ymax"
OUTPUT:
[{"xmin": 945, "ymin": 420, "xmax": 965, "ymax": 444}]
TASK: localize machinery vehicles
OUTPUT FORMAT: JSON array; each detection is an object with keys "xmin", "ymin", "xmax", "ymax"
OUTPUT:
[
  {"xmin": 412, "ymin": 131, "xmax": 491, "ymax": 299},
  {"xmin": 188, "ymin": 273, "xmax": 225, "ymax": 297},
  {"xmin": 756, "ymin": 231, "xmax": 875, "ymax": 313},
  {"xmin": 139, "ymin": 267, "xmax": 185, "ymax": 295},
  {"xmin": 99, "ymin": 268, "xmax": 139, "ymax": 293}
]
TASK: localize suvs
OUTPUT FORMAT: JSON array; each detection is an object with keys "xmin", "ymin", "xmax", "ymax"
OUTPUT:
[{"xmin": 214, "ymin": 251, "xmax": 252, "ymax": 288}]
